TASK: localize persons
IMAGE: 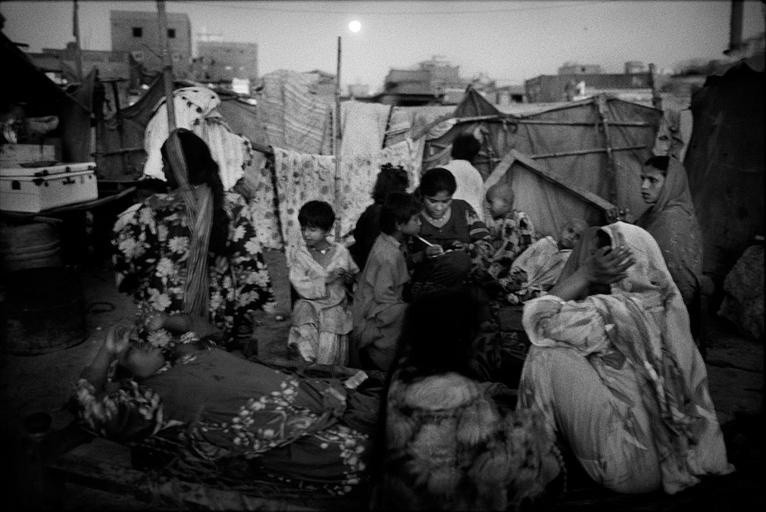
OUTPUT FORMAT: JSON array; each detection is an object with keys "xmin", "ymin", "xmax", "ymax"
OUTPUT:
[
  {"xmin": 516, "ymin": 220, "xmax": 735, "ymax": 494},
  {"xmin": 67, "ymin": 307, "xmax": 567, "ymax": 503},
  {"xmin": 108, "ymin": 128, "xmax": 276, "ymax": 349},
  {"xmin": 285, "ymin": 134, "xmax": 703, "ymax": 365}
]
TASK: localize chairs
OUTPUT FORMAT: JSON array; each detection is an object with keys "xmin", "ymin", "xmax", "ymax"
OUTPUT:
[{"xmin": 0, "ymin": 217, "xmax": 92, "ymax": 357}]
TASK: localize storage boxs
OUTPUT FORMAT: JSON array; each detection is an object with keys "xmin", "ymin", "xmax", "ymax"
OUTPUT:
[{"xmin": 0, "ymin": 158, "xmax": 98, "ymax": 215}]
